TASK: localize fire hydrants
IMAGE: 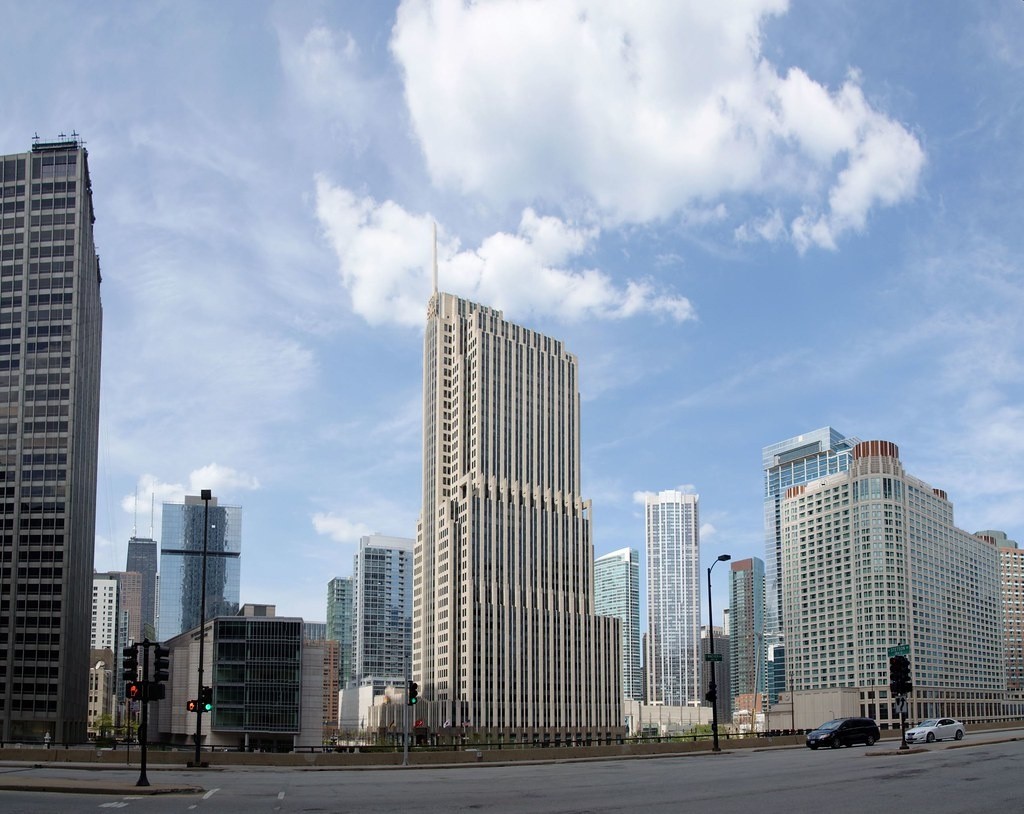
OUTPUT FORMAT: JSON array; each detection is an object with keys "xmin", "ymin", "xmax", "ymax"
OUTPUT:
[{"xmin": 43, "ymin": 733, "xmax": 51, "ymax": 750}]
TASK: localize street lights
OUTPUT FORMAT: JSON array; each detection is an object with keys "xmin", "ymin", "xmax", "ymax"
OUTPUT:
[
  {"xmin": 829, "ymin": 710, "xmax": 834, "ymax": 719},
  {"xmin": 653, "ymin": 678, "xmax": 662, "ymax": 735},
  {"xmin": 707, "ymin": 554, "xmax": 732, "ymax": 751},
  {"xmin": 870, "ymin": 678, "xmax": 875, "ymax": 719},
  {"xmin": 676, "ymin": 691, "xmax": 682, "ymax": 727},
  {"xmin": 195, "ymin": 489, "xmax": 212, "ymax": 765}
]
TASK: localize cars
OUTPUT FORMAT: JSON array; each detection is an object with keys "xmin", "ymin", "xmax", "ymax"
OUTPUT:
[
  {"xmin": 807, "ymin": 715, "xmax": 880, "ymax": 749},
  {"xmin": 904, "ymin": 716, "xmax": 966, "ymax": 744}
]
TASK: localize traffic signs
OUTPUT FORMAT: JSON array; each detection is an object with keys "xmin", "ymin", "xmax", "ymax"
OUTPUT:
[{"xmin": 702, "ymin": 654, "xmax": 724, "ymax": 662}]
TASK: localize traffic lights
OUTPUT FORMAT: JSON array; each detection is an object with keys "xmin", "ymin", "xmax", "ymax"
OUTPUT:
[
  {"xmin": 706, "ymin": 682, "xmax": 717, "ymax": 701},
  {"xmin": 125, "ymin": 681, "xmax": 144, "ymax": 700},
  {"xmin": 408, "ymin": 680, "xmax": 417, "ymax": 705},
  {"xmin": 890, "ymin": 656, "xmax": 912, "ymax": 693},
  {"xmin": 202, "ymin": 704, "xmax": 212, "ymax": 712},
  {"xmin": 187, "ymin": 700, "xmax": 197, "ymax": 712},
  {"xmin": 154, "ymin": 645, "xmax": 170, "ymax": 683},
  {"xmin": 123, "ymin": 645, "xmax": 138, "ymax": 681}
]
